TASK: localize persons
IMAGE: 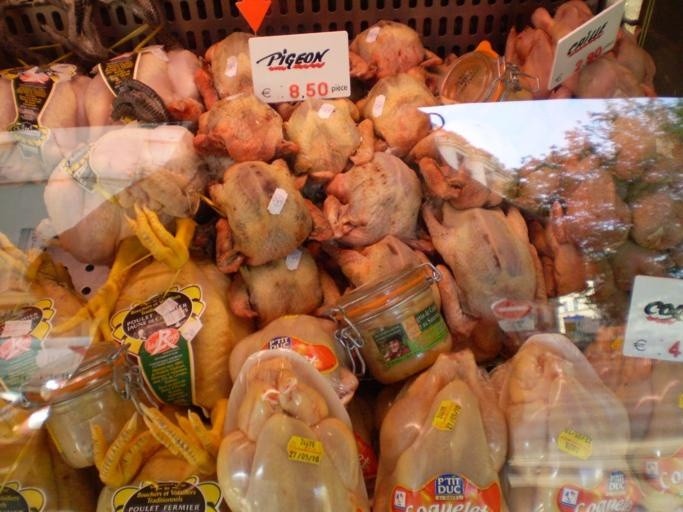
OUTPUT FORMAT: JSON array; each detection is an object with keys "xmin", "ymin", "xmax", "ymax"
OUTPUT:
[{"xmin": 384, "ymin": 333, "xmax": 411, "ymax": 362}]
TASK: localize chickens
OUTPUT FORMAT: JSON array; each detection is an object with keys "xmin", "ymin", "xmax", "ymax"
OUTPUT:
[{"xmin": 0, "ymin": 0, "xmax": 683, "ymax": 512}]
